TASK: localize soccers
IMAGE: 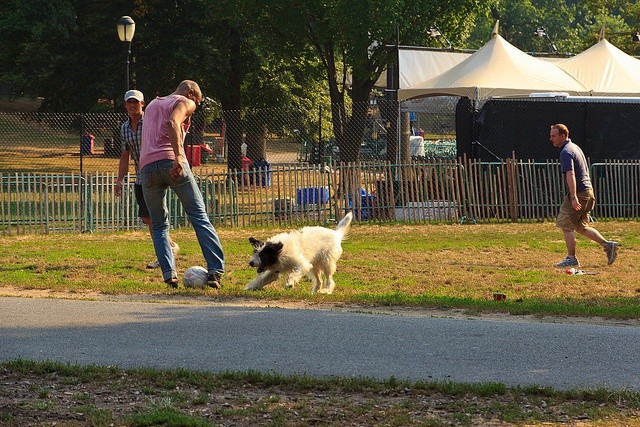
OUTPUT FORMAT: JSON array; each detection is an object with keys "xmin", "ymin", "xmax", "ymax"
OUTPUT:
[{"xmin": 183, "ymin": 265, "xmax": 209, "ymax": 289}]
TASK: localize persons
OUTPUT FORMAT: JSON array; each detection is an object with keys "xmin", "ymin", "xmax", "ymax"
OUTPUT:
[
  {"xmin": 114, "ymin": 90, "xmax": 181, "ymax": 269},
  {"xmin": 138, "ymin": 79, "xmax": 225, "ymax": 289},
  {"xmin": 549, "ymin": 123, "xmax": 619, "ymax": 268}
]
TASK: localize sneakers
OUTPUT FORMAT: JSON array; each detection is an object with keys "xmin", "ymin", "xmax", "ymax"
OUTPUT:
[
  {"xmin": 147, "ymin": 255, "xmax": 161, "ymax": 268},
  {"xmin": 207, "ymin": 274, "xmax": 221, "ymax": 288},
  {"xmin": 167, "ymin": 279, "xmax": 178, "ymax": 289},
  {"xmin": 171, "ymin": 242, "xmax": 180, "ymax": 257},
  {"xmin": 555, "ymin": 256, "xmax": 579, "ymax": 267},
  {"xmin": 603, "ymin": 243, "xmax": 619, "ymax": 265}
]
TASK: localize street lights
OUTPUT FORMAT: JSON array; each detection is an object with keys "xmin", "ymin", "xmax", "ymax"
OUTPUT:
[{"xmin": 116, "ymin": 15, "xmax": 136, "ymax": 90}]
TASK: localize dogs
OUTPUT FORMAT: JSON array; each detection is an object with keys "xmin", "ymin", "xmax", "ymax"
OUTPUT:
[{"xmin": 243, "ymin": 211, "xmax": 353, "ymax": 295}]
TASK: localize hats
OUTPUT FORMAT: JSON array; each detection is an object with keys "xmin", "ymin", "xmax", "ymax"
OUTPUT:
[{"xmin": 124, "ymin": 90, "xmax": 144, "ymax": 102}]
